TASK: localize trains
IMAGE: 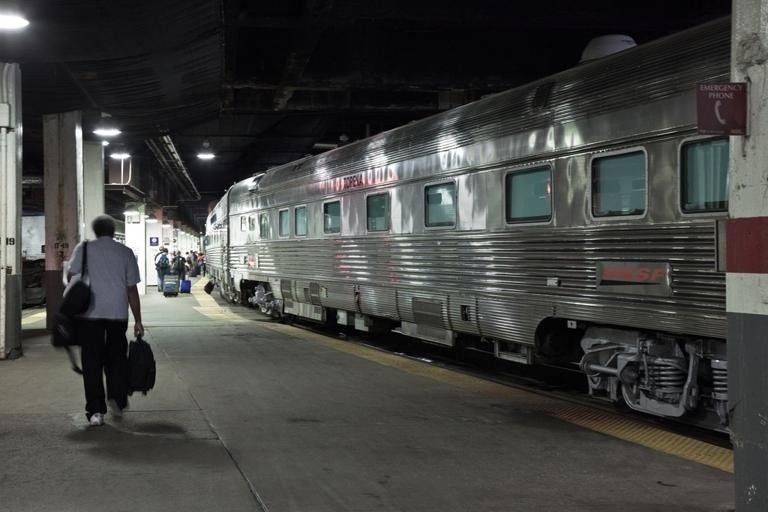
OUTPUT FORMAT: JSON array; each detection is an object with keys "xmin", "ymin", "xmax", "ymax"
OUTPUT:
[{"xmin": 203, "ymin": 14, "xmax": 732, "ymax": 431}]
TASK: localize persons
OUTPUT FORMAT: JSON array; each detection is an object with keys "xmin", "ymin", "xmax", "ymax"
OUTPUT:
[
  {"xmin": 61, "ymin": 214, "xmax": 145, "ymax": 426},
  {"xmin": 155, "ymin": 246, "xmax": 206, "ymax": 292}
]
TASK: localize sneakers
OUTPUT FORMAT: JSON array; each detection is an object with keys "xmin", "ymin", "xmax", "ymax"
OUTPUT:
[
  {"xmin": 107, "ymin": 399, "xmax": 122, "ymax": 417},
  {"xmin": 89, "ymin": 412, "xmax": 104, "ymax": 426}
]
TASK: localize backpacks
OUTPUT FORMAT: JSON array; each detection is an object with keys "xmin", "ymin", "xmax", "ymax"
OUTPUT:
[
  {"xmin": 127, "ymin": 335, "xmax": 157, "ymax": 396},
  {"xmin": 159, "ymin": 253, "xmax": 169, "ymax": 268},
  {"xmin": 176, "ymin": 259, "xmax": 184, "ymax": 271}
]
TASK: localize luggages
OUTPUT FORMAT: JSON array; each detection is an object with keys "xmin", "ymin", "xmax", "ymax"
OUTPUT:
[
  {"xmin": 204, "ymin": 281, "xmax": 214, "ymax": 294},
  {"xmin": 180, "ymin": 280, "xmax": 191, "ymax": 293},
  {"xmin": 163, "ymin": 274, "xmax": 179, "ymax": 296}
]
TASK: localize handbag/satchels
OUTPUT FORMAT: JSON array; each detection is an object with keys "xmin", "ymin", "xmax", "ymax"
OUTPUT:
[
  {"xmin": 51, "ymin": 315, "xmax": 90, "ymax": 346},
  {"xmin": 60, "ymin": 240, "xmax": 91, "ymax": 319}
]
